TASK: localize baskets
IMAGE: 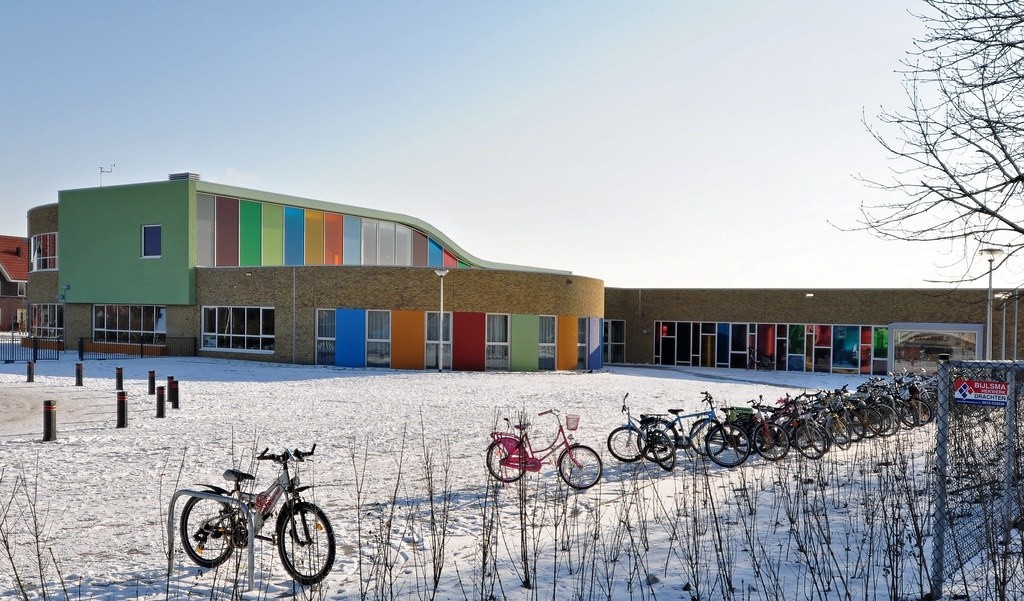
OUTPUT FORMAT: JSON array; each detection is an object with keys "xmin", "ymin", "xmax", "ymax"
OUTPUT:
[{"xmin": 567, "ymin": 413, "xmax": 580, "ymax": 430}]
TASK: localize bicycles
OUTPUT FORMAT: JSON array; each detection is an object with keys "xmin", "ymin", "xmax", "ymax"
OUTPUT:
[
  {"xmin": 483, "ymin": 410, "xmax": 603, "ymax": 490},
  {"xmin": 720, "ymin": 367, "xmax": 956, "ymax": 462},
  {"xmin": 637, "ymin": 391, "xmax": 751, "ymax": 468},
  {"xmin": 179, "ymin": 443, "xmax": 336, "ymax": 586},
  {"xmin": 607, "ymin": 392, "xmax": 676, "ymax": 471}
]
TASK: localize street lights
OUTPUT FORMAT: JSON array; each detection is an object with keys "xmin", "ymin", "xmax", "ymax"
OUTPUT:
[
  {"xmin": 435, "ymin": 269, "xmax": 449, "ymax": 372},
  {"xmin": 979, "ymin": 249, "xmax": 1004, "ymax": 423}
]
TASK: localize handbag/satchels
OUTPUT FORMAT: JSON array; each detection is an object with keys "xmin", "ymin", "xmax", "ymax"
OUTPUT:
[{"xmin": 730, "ymin": 407, "xmax": 753, "ymax": 425}]
